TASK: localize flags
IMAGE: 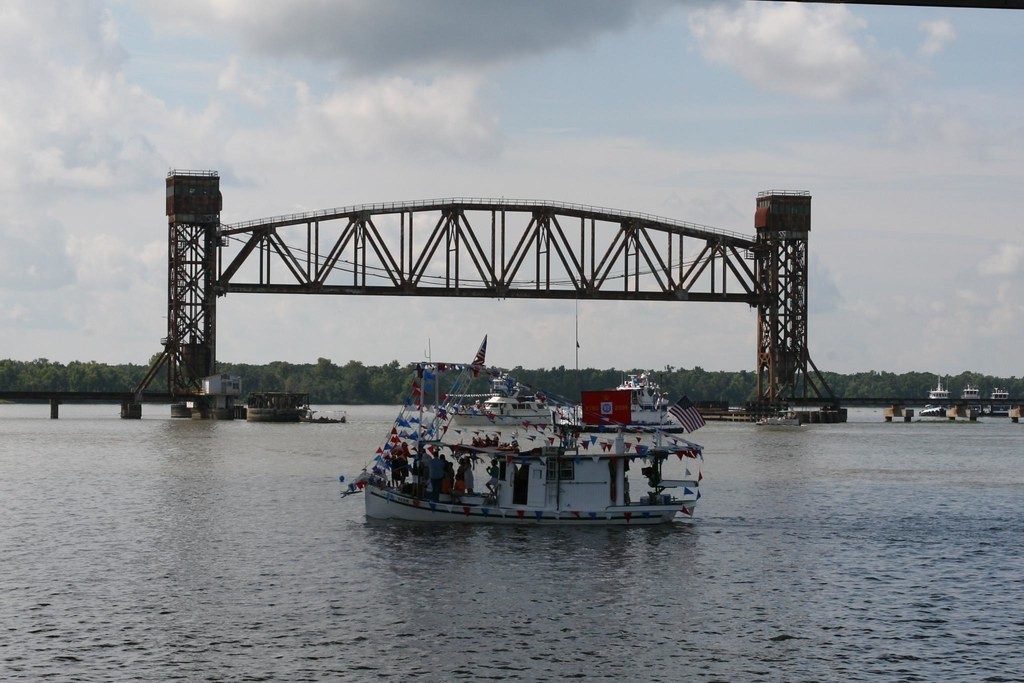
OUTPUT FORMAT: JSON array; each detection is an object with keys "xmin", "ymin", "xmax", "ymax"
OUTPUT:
[
  {"xmin": 669, "ymin": 395, "xmax": 706, "ymax": 434},
  {"xmin": 472, "ymin": 335, "xmax": 487, "ymax": 377}
]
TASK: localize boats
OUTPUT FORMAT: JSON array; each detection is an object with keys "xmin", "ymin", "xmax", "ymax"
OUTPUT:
[
  {"xmin": 961, "ymin": 384, "xmax": 983, "ymax": 414},
  {"xmin": 296, "ymin": 414, "xmax": 344, "ymax": 423},
  {"xmin": 983, "ymin": 386, "xmax": 1013, "ymax": 416},
  {"xmin": 560, "ymin": 370, "xmax": 685, "ymax": 433},
  {"xmin": 919, "ymin": 373, "xmax": 952, "ymax": 416},
  {"xmin": 755, "ymin": 409, "xmax": 800, "ymax": 427},
  {"xmin": 339, "ymin": 333, "xmax": 708, "ymax": 526},
  {"xmin": 244, "ymin": 389, "xmax": 310, "ymax": 422}
]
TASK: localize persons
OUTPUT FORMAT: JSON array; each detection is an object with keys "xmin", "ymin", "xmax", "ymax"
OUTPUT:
[{"xmin": 391, "ymin": 442, "xmax": 500, "ymax": 504}]
